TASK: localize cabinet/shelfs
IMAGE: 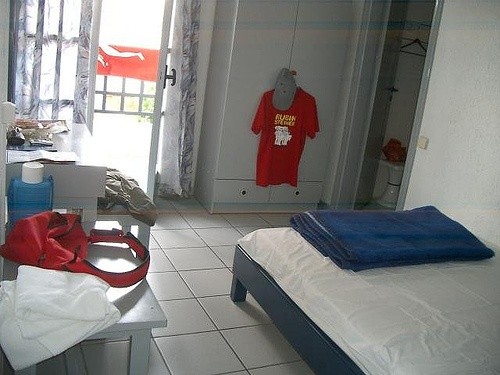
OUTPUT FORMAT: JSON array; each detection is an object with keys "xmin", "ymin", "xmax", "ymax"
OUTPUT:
[{"xmin": 193, "ymin": 0, "xmax": 361, "ymax": 214}]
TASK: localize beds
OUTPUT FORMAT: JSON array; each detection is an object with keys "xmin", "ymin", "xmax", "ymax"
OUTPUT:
[{"xmin": 231, "ymin": 226, "xmax": 500, "ymax": 375}]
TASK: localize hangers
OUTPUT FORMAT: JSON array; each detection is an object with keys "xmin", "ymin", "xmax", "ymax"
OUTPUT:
[{"xmin": 392, "ymin": 37, "xmax": 427, "ymax": 58}]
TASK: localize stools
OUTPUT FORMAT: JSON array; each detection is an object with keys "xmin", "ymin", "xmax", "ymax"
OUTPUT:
[{"xmin": 71, "ymin": 205, "xmax": 150, "ymax": 266}]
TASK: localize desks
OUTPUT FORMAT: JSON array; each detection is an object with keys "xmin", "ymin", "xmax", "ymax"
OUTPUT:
[
  {"xmin": 0, "ymin": 235, "xmax": 167, "ymax": 375},
  {"xmin": 5, "ymin": 123, "xmax": 107, "ymax": 222}
]
